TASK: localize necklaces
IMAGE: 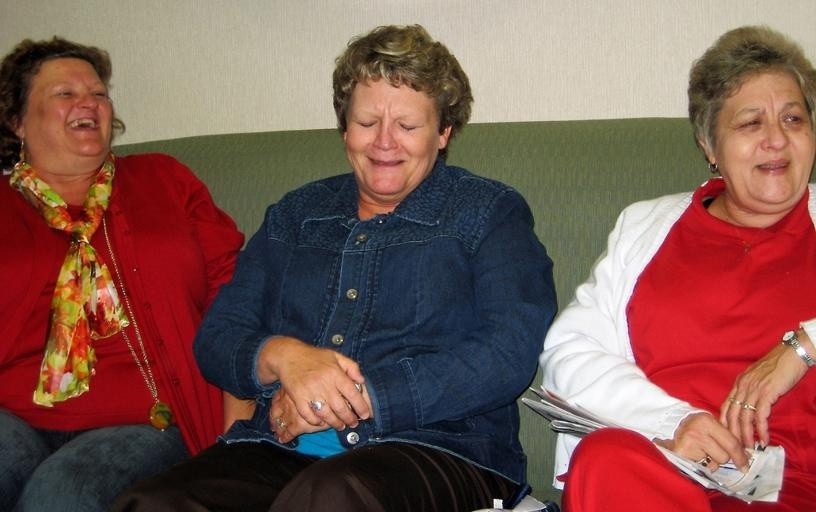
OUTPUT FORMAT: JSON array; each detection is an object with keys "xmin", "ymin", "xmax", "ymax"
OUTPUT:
[{"xmin": 71, "ymin": 223, "xmax": 175, "ymax": 437}]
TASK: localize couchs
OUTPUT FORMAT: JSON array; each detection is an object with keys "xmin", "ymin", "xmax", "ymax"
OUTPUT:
[{"xmin": 112, "ymin": 117, "xmax": 718, "ymax": 510}]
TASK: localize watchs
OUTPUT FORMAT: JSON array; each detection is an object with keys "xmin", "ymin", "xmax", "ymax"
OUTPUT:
[{"xmin": 782, "ymin": 330, "xmax": 815, "ymax": 368}]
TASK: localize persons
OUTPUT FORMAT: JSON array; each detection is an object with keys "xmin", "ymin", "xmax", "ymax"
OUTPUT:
[
  {"xmin": 0, "ymin": 32, "xmax": 258, "ymax": 512},
  {"xmin": 534, "ymin": 21, "xmax": 814, "ymax": 511},
  {"xmin": 103, "ymin": 23, "xmax": 562, "ymax": 512}
]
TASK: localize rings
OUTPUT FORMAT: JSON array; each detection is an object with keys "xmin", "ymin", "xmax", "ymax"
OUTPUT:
[
  {"xmin": 309, "ymin": 398, "xmax": 326, "ymax": 413},
  {"xmin": 729, "ymin": 397, "xmax": 735, "ymax": 402},
  {"xmin": 693, "ymin": 455, "xmax": 712, "ymax": 466},
  {"xmin": 274, "ymin": 415, "xmax": 288, "ymax": 429},
  {"xmin": 733, "ymin": 399, "xmax": 743, "ymax": 406},
  {"xmin": 743, "ymin": 402, "xmax": 757, "ymax": 410}
]
